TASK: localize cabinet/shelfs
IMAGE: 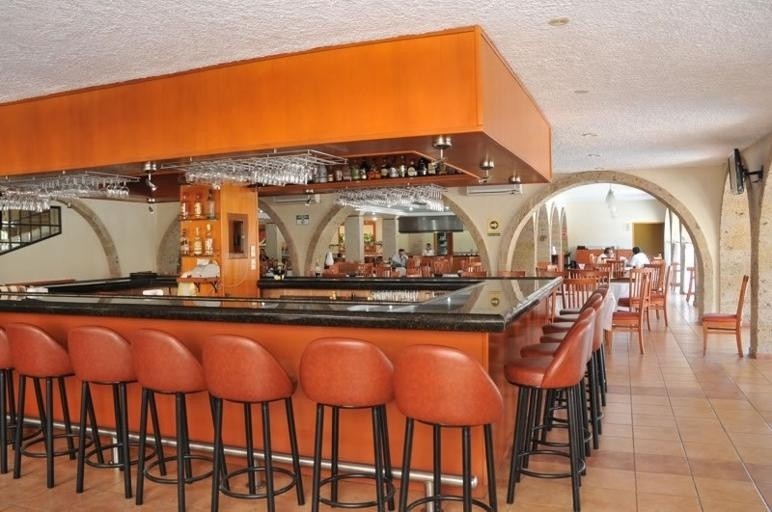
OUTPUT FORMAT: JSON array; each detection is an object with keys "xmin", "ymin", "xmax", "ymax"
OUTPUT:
[{"xmin": 176, "ymin": 184, "xmax": 260, "ymax": 298}]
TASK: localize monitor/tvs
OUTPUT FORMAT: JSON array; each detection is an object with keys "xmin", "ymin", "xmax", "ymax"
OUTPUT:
[{"xmin": 727, "ymin": 148, "xmax": 747, "ymax": 195}]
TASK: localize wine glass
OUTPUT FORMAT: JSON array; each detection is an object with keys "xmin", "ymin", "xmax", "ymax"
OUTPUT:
[
  {"xmin": 334, "ymin": 185, "xmax": 444, "ymax": 207},
  {"xmin": 0, "ymin": 174, "xmax": 130, "ymax": 213},
  {"xmin": 183, "ymin": 154, "xmax": 310, "ymax": 188},
  {"xmin": 358, "ymin": 263, "xmax": 365, "ymax": 277}
]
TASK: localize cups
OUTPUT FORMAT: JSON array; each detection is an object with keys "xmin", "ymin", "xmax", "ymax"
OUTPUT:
[{"xmin": 578, "ymin": 263, "xmax": 585, "ymax": 269}]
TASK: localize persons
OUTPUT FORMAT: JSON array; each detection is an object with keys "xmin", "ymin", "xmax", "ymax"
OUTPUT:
[
  {"xmin": 423, "ymin": 243, "xmax": 434, "ymax": 256},
  {"xmin": 597, "ymin": 247, "xmax": 615, "ymax": 264},
  {"xmin": 392, "ymin": 248, "xmax": 409, "ymax": 277},
  {"xmin": 626, "ymin": 246, "xmax": 650, "ymax": 269}
]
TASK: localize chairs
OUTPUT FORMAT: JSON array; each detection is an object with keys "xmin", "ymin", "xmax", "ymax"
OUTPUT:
[
  {"xmin": 701, "ymin": 275, "xmax": 749, "ymax": 358},
  {"xmin": 321, "ymin": 256, "xmax": 487, "ymax": 278}
]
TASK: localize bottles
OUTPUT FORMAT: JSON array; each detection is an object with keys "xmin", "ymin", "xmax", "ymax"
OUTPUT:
[
  {"xmin": 180, "ymin": 187, "xmax": 216, "ymax": 218},
  {"xmin": 309, "ymin": 157, "xmax": 463, "ymax": 185},
  {"xmin": 274, "ymin": 263, "xmax": 285, "ymax": 281},
  {"xmin": 181, "ymin": 224, "xmax": 215, "ymax": 256}
]
